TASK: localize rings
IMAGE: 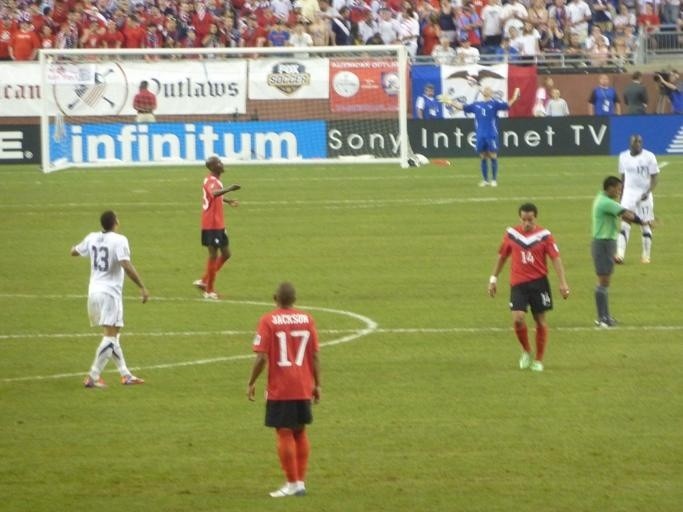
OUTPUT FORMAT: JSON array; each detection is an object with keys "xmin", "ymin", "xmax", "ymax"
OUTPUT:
[{"xmin": 566, "ymin": 290, "xmax": 569, "ymax": 294}]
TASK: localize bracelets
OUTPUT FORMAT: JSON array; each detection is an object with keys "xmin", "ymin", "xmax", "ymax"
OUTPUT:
[{"xmin": 489, "ymin": 275, "xmax": 497, "ymax": 284}]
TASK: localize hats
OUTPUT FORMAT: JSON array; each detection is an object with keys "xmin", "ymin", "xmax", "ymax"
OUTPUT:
[{"xmin": 0, "ymin": 0, "xmax": 683, "ymax": 45}]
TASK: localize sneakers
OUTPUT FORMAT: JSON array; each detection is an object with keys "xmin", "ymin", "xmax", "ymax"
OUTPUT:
[
  {"xmin": 119, "ymin": 374, "xmax": 145, "ymax": 385},
  {"xmin": 269, "ymin": 480, "xmax": 306, "ymax": 497},
  {"xmin": 641, "ymin": 258, "xmax": 651, "ymax": 265},
  {"xmin": 532, "ymin": 361, "xmax": 543, "ymax": 372},
  {"xmin": 479, "ymin": 181, "xmax": 497, "ymax": 188},
  {"xmin": 615, "ymin": 255, "xmax": 626, "ymax": 263},
  {"xmin": 519, "ymin": 352, "xmax": 530, "ymax": 369},
  {"xmin": 204, "ymin": 292, "xmax": 224, "ymax": 300},
  {"xmin": 83, "ymin": 375, "xmax": 109, "ymax": 388},
  {"xmin": 193, "ymin": 279, "xmax": 207, "ymax": 289},
  {"xmin": 594, "ymin": 317, "xmax": 617, "ymax": 327}
]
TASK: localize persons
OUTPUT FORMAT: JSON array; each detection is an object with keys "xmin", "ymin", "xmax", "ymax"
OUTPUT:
[
  {"xmin": 613, "ymin": 132, "xmax": 661, "ymax": 265},
  {"xmin": 133, "ymin": 81, "xmax": 157, "ymax": 121},
  {"xmin": 69, "ymin": 210, "xmax": 148, "ymax": 389},
  {"xmin": 587, "ymin": 74, "xmax": 623, "ymax": 117},
  {"xmin": 245, "ymin": 281, "xmax": 320, "ymax": 498},
  {"xmin": 531, "ymin": 77, "xmax": 569, "ymax": 118},
  {"xmin": 624, "ymin": 71, "xmax": 649, "ymax": 114},
  {"xmin": 448, "ymin": 84, "xmax": 521, "ymax": 187},
  {"xmin": 193, "ymin": 156, "xmax": 241, "ymax": 302},
  {"xmin": 655, "ymin": 68, "xmax": 683, "ymax": 115},
  {"xmin": 1, "ymin": 0, "xmax": 682, "ymax": 67},
  {"xmin": 415, "ymin": 84, "xmax": 445, "ymax": 120},
  {"xmin": 486, "ymin": 202, "xmax": 570, "ymax": 372},
  {"xmin": 589, "ymin": 175, "xmax": 650, "ymax": 330}
]
